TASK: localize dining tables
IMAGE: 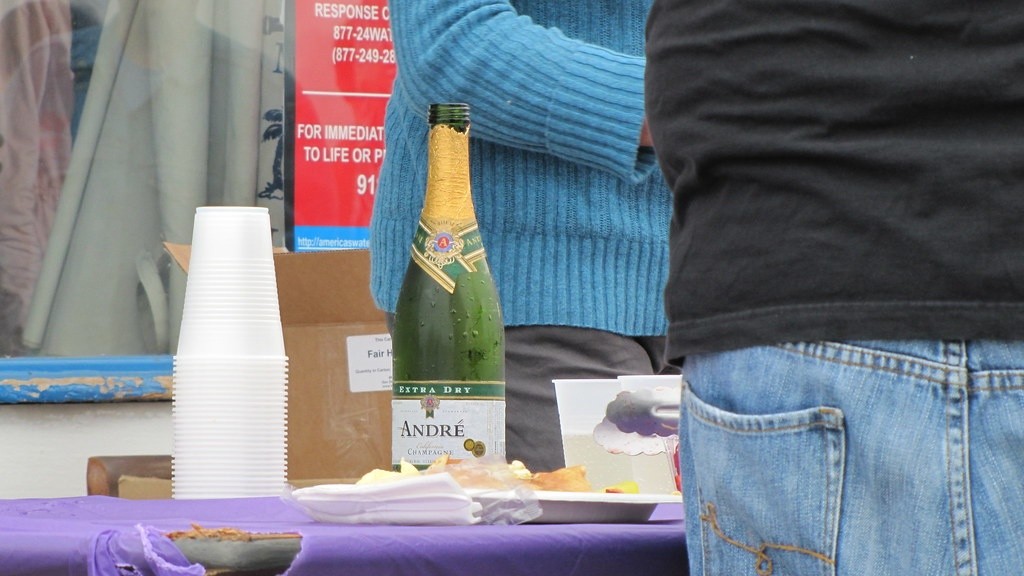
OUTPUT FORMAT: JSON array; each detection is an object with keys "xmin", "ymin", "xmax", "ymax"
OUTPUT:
[{"xmin": 0, "ymin": 494, "xmax": 692, "ymax": 575}]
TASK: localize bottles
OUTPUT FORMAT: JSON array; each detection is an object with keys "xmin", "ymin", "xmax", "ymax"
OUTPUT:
[{"xmin": 393, "ymin": 104, "xmax": 508, "ymax": 470}]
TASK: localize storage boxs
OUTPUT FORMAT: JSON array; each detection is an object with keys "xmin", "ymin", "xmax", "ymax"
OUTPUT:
[
  {"xmin": 162, "ymin": 235, "xmax": 392, "ymax": 489},
  {"xmin": 84, "ymin": 454, "xmax": 172, "ymax": 499}
]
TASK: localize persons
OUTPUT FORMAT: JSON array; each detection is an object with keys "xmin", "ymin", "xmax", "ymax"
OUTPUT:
[
  {"xmin": 371, "ymin": 0, "xmax": 687, "ymax": 473},
  {"xmin": 643, "ymin": 0, "xmax": 1022, "ymax": 575}
]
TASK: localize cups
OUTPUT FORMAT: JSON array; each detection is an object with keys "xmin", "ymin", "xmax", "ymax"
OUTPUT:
[
  {"xmin": 172, "ymin": 208, "xmax": 291, "ymax": 500},
  {"xmin": 620, "ymin": 376, "xmax": 685, "ymax": 496},
  {"xmin": 550, "ymin": 379, "xmax": 632, "ymax": 493}
]
